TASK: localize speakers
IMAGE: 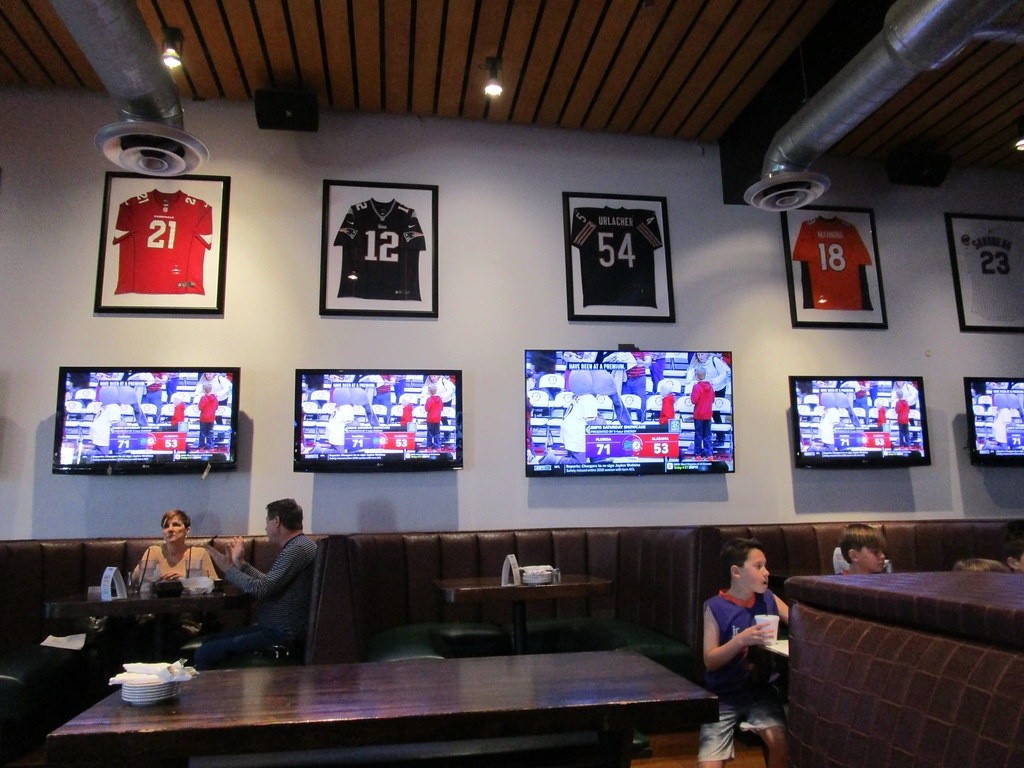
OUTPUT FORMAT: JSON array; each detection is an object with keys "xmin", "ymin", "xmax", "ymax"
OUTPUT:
[
  {"xmin": 255, "ymin": 88, "xmax": 319, "ymax": 132},
  {"xmin": 885, "ymin": 145, "xmax": 952, "ymax": 188}
]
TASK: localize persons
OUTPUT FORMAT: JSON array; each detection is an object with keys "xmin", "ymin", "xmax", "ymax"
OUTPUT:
[
  {"xmin": 796, "ymin": 380, "xmax": 918, "ymax": 451},
  {"xmin": 303, "ymin": 375, "xmax": 455, "ymax": 454},
  {"xmin": 525, "ymin": 351, "xmax": 731, "ymax": 464},
  {"xmin": 837, "ymin": 524, "xmax": 888, "ymax": 576},
  {"xmin": 972, "ymin": 381, "xmax": 1024, "ymax": 450},
  {"xmin": 130, "ymin": 510, "xmax": 220, "ymax": 591},
  {"xmin": 698, "ymin": 537, "xmax": 790, "ymax": 768},
  {"xmin": 193, "ymin": 499, "xmax": 318, "ymax": 671},
  {"xmin": 63, "ymin": 371, "xmax": 233, "ymax": 456},
  {"xmin": 951, "ymin": 520, "xmax": 1024, "ymax": 574}
]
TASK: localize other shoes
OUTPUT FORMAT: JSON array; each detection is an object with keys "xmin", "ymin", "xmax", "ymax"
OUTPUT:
[
  {"xmin": 425, "ymin": 447, "xmax": 432, "ymax": 451},
  {"xmin": 197, "ymin": 449, "xmax": 205, "ymax": 453},
  {"xmin": 209, "ymin": 448, "xmax": 215, "ymax": 453},
  {"xmin": 900, "ymin": 445, "xmax": 911, "ymax": 450},
  {"xmin": 692, "ymin": 455, "xmax": 700, "ymax": 461},
  {"xmin": 705, "ymin": 455, "xmax": 713, "ymax": 461},
  {"xmin": 437, "ymin": 448, "xmax": 441, "ymax": 452}
]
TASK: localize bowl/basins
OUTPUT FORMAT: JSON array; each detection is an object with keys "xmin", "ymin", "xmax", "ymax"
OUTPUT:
[{"xmin": 151, "ymin": 582, "xmax": 183, "ymax": 597}]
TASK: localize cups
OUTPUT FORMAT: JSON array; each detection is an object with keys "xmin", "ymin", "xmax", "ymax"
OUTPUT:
[
  {"xmin": 755, "ymin": 614, "xmax": 780, "ymax": 644},
  {"xmin": 139, "ymin": 560, "xmax": 155, "ymax": 593},
  {"xmin": 185, "ymin": 559, "xmax": 202, "ymax": 578},
  {"xmin": 212, "ymin": 579, "xmax": 223, "ymax": 593},
  {"xmin": 125, "ymin": 571, "xmax": 134, "ymax": 594}
]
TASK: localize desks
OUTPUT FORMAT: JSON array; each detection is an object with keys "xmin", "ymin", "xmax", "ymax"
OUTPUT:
[
  {"xmin": 45, "ymin": 647, "xmax": 721, "ymax": 768},
  {"xmin": 43, "ymin": 583, "xmax": 247, "ymax": 698},
  {"xmin": 432, "ymin": 571, "xmax": 612, "ymax": 656}
]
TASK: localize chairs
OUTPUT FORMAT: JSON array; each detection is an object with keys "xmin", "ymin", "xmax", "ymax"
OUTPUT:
[
  {"xmin": 527, "ymin": 353, "xmax": 732, "ymax": 453},
  {"xmin": 798, "ymin": 380, "xmax": 922, "ymax": 448},
  {"xmin": 62, "ymin": 373, "xmax": 231, "ymax": 444},
  {"xmin": 302, "ymin": 375, "xmax": 456, "ymax": 445},
  {"xmin": 972, "ymin": 382, "xmax": 1024, "ymax": 447}
]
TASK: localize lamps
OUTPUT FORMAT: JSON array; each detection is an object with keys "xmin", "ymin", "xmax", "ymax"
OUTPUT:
[
  {"xmin": 481, "ymin": 56, "xmax": 504, "ymax": 97},
  {"xmin": 160, "ymin": 26, "xmax": 185, "ymax": 68}
]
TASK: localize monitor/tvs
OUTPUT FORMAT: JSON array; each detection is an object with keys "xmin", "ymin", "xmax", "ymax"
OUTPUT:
[
  {"xmin": 294, "ymin": 369, "xmax": 464, "ymax": 474},
  {"xmin": 524, "ymin": 350, "xmax": 735, "ymax": 477},
  {"xmin": 963, "ymin": 377, "xmax": 1024, "ymax": 465},
  {"xmin": 52, "ymin": 367, "xmax": 241, "ymax": 475},
  {"xmin": 788, "ymin": 376, "xmax": 931, "ymax": 469}
]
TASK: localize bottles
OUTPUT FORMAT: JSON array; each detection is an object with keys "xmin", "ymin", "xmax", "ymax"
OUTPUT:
[{"xmin": 553, "ymin": 568, "xmax": 562, "ymax": 582}]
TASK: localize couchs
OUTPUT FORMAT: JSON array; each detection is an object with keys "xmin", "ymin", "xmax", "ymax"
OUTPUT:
[{"xmin": 0, "ymin": 518, "xmax": 1023, "ymax": 768}]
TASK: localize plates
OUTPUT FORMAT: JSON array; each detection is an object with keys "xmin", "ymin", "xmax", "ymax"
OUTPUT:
[
  {"xmin": 522, "ymin": 572, "xmax": 552, "ymax": 583},
  {"xmin": 121, "ymin": 680, "xmax": 180, "ymax": 706}
]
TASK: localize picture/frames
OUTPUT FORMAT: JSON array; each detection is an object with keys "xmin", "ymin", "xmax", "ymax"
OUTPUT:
[
  {"xmin": 93, "ymin": 170, "xmax": 231, "ymax": 316},
  {"xmin": 780, "ymin": 204, "xmax": 889, "ymax": 330},
  {"xmin": 560, "ymin": 191, "xmax": 677, "ymax": 323},
  {"xmin": 318, "ymin": 180, "xmax": 439, "ymax": 319},
  {"xmin": 943, "ymin": 211, "xmax": 1024, "ymax": 333}
]
